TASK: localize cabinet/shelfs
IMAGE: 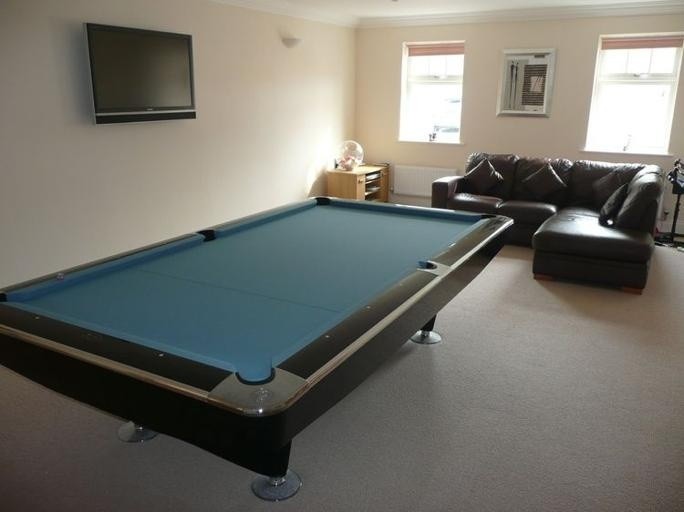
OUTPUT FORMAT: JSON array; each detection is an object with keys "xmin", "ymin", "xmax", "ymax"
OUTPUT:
[{"xmin": 326, "ymin": 162, "xmax": 390, "ymax": 202}]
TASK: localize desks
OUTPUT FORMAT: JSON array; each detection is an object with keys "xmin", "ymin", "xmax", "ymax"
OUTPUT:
[{"xmin": 0, "ymin": 196, "xmax": 514, "ymax": 502}]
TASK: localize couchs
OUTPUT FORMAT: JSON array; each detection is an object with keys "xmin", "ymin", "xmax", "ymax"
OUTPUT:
[{"xmin": 432, "ymin": 152, "xmax": 666, "ymax": 295}]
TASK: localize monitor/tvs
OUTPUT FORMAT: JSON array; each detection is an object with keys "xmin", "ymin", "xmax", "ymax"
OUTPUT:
[{"xmin": 87, "ymin": 23, "xmax": 196, "ymax": 124}]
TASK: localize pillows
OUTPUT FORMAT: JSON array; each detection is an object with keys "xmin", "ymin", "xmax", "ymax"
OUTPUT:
[
  {"xmin": 587, "ymin": 168, "xmax": 622, "ymax": 209},
  {"xmin": 521, "ymin": 163, "xmax": 568, "ymax": 200},
  {"xmin": 599, "ymin": 183, "xmax": 629, "ymax": 228},
  {"xmin": 464, "ymin": 158, "xmax": 504, "ymax": 195}
]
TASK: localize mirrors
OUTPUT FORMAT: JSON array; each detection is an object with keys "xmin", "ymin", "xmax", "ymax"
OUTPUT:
[{"xmin": 495, "ymin": 46, "xmax": 558, "ymax": 119}]
